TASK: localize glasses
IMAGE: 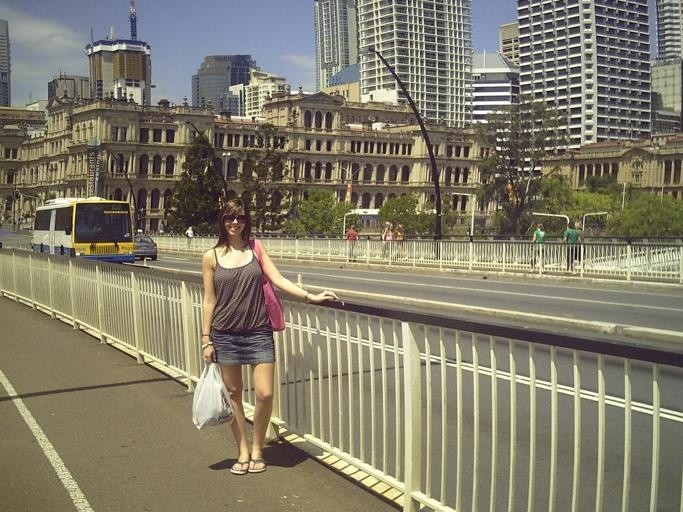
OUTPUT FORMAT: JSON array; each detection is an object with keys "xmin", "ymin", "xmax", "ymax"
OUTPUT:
[{"xmin": 224, "ymin": 214, "xmax": 249, "ymax": 223}]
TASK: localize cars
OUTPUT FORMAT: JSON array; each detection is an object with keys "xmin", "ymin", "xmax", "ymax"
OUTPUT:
[{"xmin": 130, "ymin": 234, "xmax": 158, "ymax": 262}]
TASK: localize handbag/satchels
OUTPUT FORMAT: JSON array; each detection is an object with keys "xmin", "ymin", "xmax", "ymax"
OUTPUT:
[{"xmin": 263, "ymin": 278, "xmax": 285, "ymax": 331}]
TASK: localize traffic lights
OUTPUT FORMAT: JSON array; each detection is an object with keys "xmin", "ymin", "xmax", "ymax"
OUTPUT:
[{"xmin": 14, "ymin": 190, "xmax": 20, "ymax": 200}]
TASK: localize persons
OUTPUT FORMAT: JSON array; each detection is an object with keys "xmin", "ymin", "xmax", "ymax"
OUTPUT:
[
  {"xmin": 531, "ymin": 223, "xmax": 547, "ymax": 275},
  {"xmin": 575, "ymin": 220, "xmax": 588, "ymax": 270},
  {"xmin": 183, "ymin": 226, "xmax": 195, "ymax": 251},
  {"xmin": 342, "ymin": 224, "xmax": 359, "ymax": 262},
  {"xmin": 159, "ymin": 220, "xmax": 167, "ymax": 237},
  {"xmin": 379, "ymin": 219, "xmax": 408, "ymax": 261},
  {"xmin": 562, "ymin": 221, "xmax": 583, "ymax": 272},
  {"xmin": 199, "ymin": 197, "xmax": 340, "ymax": 476}
]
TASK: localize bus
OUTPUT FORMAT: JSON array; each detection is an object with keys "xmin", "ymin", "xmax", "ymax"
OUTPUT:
[
  {"xmin": 30, "ymin": 196, "xmax": 135, "ymax": 271},
  {"xmin": 336, "ymin": 208, "xmax": 442, "ymax": 240}
]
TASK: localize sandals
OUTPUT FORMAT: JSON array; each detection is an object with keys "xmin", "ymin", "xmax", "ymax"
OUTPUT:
[
  {"xmin": 230, "ymin": 456, "xmax": 249, "ymax": 475},
  {"xmin": 249, "ymin": 452, "xmax": 267, "ymax": 473}
]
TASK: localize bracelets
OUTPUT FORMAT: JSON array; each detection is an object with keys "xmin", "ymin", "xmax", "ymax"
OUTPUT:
[
  {"xmin": 201, "ymin": 334, "xmax": 211, "ymax": 337},
  {"xmin": 200, "ymin": 341, "xmax": 215, "ymax": 351},
  {"xmin": 303, "ymin": 290, "xmax": 312, "ymax": 302}
]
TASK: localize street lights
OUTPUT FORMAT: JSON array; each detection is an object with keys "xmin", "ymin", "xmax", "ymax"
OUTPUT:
[
  {"xmin": 338, "ymin": 165, "xmax": 367, "ymax": 206},
  {"xmin": 180, "ymin": 118, "xmax": 228, "ymax": 208},
  {"xmin": 621, "ymin": 180, "xmax": 627, "ymax": 212},
  {"xmin": 12, "ymin": 167, "xmax": 18, "ymax": 226},
  {"xmin": 93, "ymin": 140, "xmax": 141, "ymax": 237},
  {"xmin": 582, "ymin": 210, "xmax": 608, "ymax": 238},
  {"xmin": 357, "ymin": 45, "xmax": 444, "ymax": 244}
]
